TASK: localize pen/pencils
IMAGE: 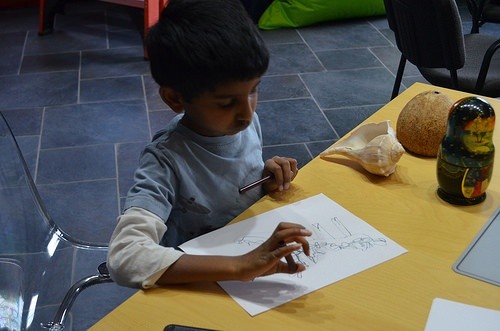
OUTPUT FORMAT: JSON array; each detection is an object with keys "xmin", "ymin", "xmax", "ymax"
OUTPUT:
[{"xmin": 239, "ymin": 162, "xmax": 305, "ymax": 194}]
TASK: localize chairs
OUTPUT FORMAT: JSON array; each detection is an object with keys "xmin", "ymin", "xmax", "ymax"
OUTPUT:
[
  {"xmin": 0, "ymin": 109, "xmax": 139, "ymax": 331},
  {"xmin": 383, "ymin": 0, "xmax": 500, "ymax": 101}
]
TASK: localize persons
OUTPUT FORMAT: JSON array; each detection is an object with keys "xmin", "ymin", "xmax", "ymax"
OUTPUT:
[{"xmin": 106, "ymin": 0, "xmax": 312, "ymax": 290}]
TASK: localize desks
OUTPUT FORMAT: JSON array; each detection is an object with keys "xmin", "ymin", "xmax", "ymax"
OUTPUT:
[{"xmin": 86, "ymin": 81, "xmax": 500, "ymax": 331}]
